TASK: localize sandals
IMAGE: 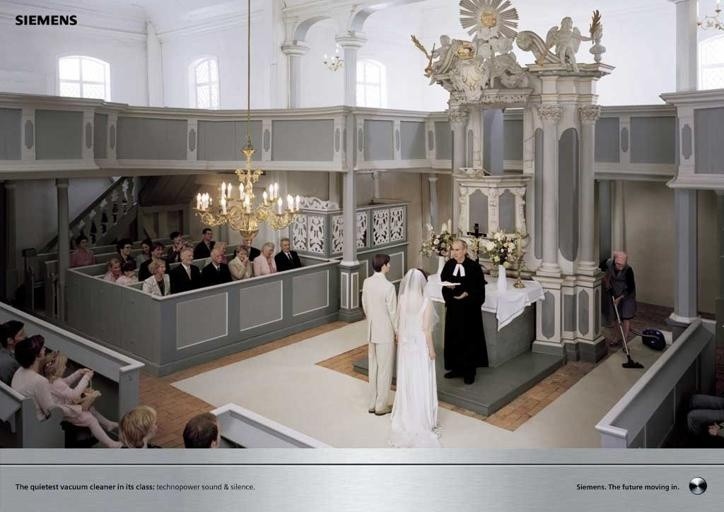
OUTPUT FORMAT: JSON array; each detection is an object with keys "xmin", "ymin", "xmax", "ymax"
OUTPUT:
[
  {"xmin": 622, "ymin": 346, "xmax": 630, "ymax": 353},
  {"xmin": 609, "ymin": 338, "xmax": 622, "ymax": 347}
]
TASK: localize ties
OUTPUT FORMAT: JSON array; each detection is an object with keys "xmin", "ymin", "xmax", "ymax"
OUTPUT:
[{"xmin": 288, "ymin": 254, "xmax": 293, "ymax": 263}]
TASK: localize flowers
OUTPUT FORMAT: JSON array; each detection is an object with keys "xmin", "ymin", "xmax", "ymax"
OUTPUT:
[
  {"xmin": 418, "ymin": 220, "xmax": 456, "ymax": 257},
  {"xmin": 460, "ymin": 236, "xmax": 479, "ymax": 264},
  {"xmin": 484, "ymin": 227, "xmax": 517, "ymax": 267}
]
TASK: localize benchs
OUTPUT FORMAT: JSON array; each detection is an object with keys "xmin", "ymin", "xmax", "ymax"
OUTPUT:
[
  {"xmin": 39, "ymin": 233, "xmax": 273, "ymax": 289},
  {"xmin": 1, "ymin": 381, "xmax": 67, "ymax": 448}
]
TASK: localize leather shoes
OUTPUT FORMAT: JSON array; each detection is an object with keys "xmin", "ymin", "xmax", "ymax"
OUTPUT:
[
  {"xmin": 369, "ymin": 408, "xmax": 375, "ymax": 413},
  {"xmin": 444, "ymin": 371, "xmax": 459, "ymax": 379},
  {"xmin": 375, "ymin": 405, "xmax": 393, "ymax": 415},
  {"xmin": 464, "ymin": 372, "xmax": 476, "ymax": 385}
]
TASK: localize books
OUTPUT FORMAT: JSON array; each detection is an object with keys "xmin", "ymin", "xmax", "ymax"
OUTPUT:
[{"xmin": 442, "ymin": 282, "xmax": 461, "ymax": 286}]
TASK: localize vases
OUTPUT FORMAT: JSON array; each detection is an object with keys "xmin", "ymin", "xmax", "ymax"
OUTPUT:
[
  {"xmin": 495, "ymin": 266, "xmax": 510, "ymax": 293},
  {"xmin": 437, "ymin": 255, "xmax": 447, "ymax": 282}
]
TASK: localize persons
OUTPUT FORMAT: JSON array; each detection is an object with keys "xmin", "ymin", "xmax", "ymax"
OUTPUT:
[
  {"xmin": 546, "ymin": 17, "xmax": 592, "ymax": 73},
  {"xmin": 599, "ymin": 251, "xmax": 636, "ymax": 354},
  {"xmin": 119, "ymin": 406, "xmax": 162, "ymax": 448},
  {"xmin": 441, "ymin": 239, "xmax": 488, "ymax": 386},
  {"xmin": 687, "ymin": 394, "xmax": 723, "ymax": 436},
  {"xmin": 361, "ymin": 255, "xmax": 399, "ymax": 416},
  {"xmin": 390, "ymin": 268, "xmax": 440, "ymax": 448},
  {"xmin": 1, "ymin": 320, "xmax": 27, "ymax": 387},
  {"xmin": 42, "ymin": 350, "xmax": 125, "ymax": 448},
  {"xmin": 13, "ymin": 333, "xmax": 82, "ymax": 421},
  {"xmin": 69, "ymin": 227, "xmax": 302, "ymax": 296},
  {"xmin": 183, "ymin": 412, "xmax": 221, "ymax": 447},
  {"xmin": 428, "ymin": 35, "xmax": 450, "ymax": 87}
]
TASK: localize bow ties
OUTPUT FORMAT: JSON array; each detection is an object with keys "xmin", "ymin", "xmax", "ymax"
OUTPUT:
[{"xmin": 453, "ymin": 263, "xmax": 465, "ymax": 277}]
